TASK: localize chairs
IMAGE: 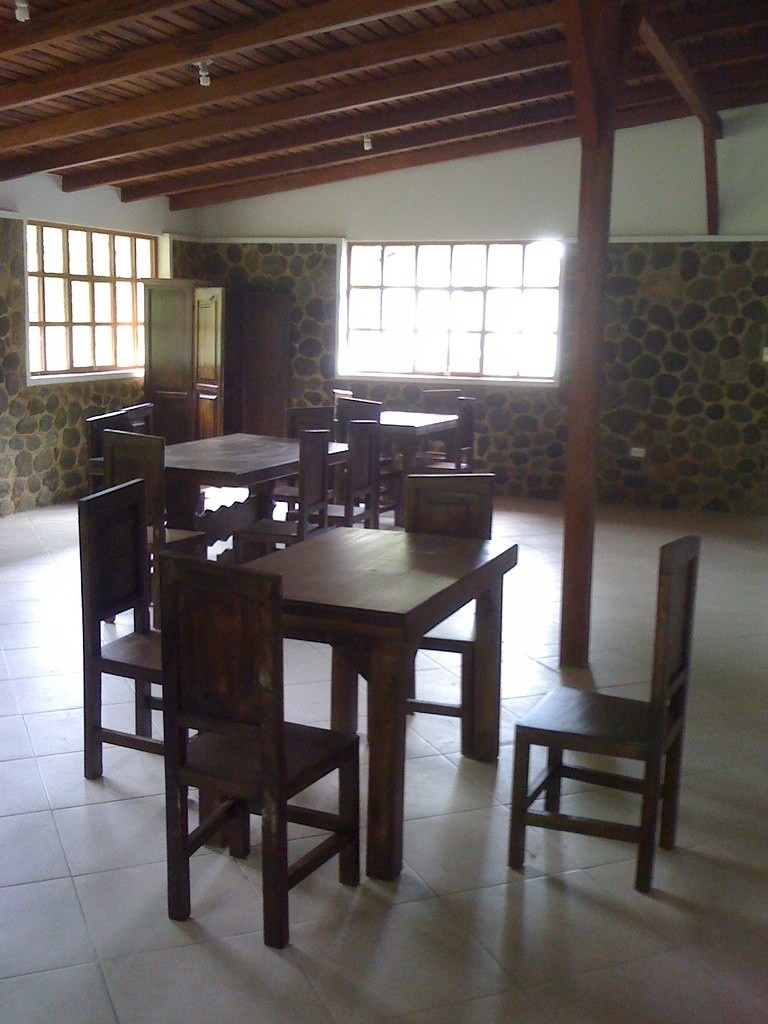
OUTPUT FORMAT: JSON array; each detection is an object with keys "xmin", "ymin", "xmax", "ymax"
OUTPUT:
[
  {"xmin": 505, "ymin": 536, "xmax": 701, "ymax": 894},
  {"xmin": 158, "ymin": 551, "xmax": 360, "ymax": 950},
  {"xmin": 234, "ymin": 387, "xmax": 476, "ymax": 565},
  {"xmin": 86, "ymin": 403, "xmax": 207, "ymax": 630},
  {"xmin": 399, "ymin": 473, "xmax": 495, "ymax": 758},
  {"xmin": 78, "ymin": 478, "xmax": 167, "ymax": 780}
]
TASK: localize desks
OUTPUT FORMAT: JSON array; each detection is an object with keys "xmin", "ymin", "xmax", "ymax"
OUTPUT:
[
  {"xmin": 378, "ymin": 410, "xmax": 459, "ymax": 527},
  {"xmin": 87, "ymin": 432, "xmax": 349, "ymax": 563},
  {"xmin": 198, "ymin": 526, "xmax": 518, "ymax": 882}
]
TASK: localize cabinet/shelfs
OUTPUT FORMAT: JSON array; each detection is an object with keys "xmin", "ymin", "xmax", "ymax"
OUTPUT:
[{"xmin": 138, "ymin": 278, "xmax": 291, "ymax": 448}]
